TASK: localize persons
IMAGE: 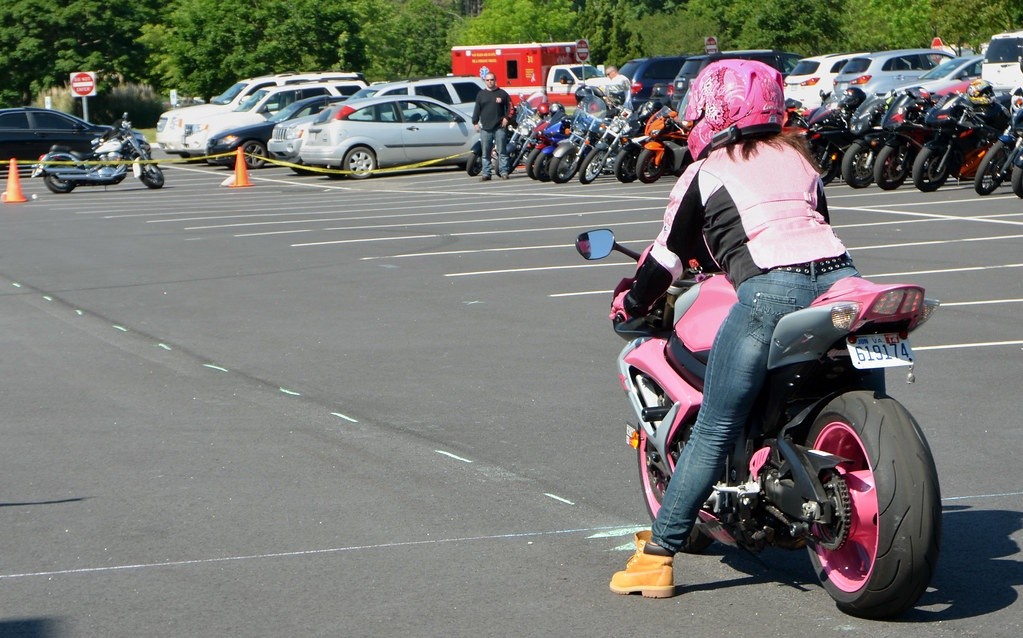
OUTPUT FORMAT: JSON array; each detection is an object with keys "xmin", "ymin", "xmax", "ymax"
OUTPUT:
[
  {"xmin": 610, "ymin": 59, "xmax": 862, "ymax": 599},
  {"xmin": 603, "ymin": 66, "xmax": 634, "ymax": 111},
  {"xmin": 472, "ymin": 72, "xmax": 514, "ymax": 181}
]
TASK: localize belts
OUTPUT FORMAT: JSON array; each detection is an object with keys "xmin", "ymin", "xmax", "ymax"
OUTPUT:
[{"xmin": 772, "ymin": 256, "xmax": 855, "ymax": 274}]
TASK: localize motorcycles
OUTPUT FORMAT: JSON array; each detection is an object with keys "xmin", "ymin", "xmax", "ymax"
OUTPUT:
[
  {"xmin": 30, "ymin": 111, "xmax": 165, "ymax": 194},
  {"xmin": 465, "ymin": 84, "xmax": 1022, "ymax": 199},
  {"xmin": 575, "ymin": 228, "xmax": 945, "ymax": 625}
]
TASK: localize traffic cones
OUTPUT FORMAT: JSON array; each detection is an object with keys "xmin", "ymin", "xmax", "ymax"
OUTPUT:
[
  {"xmin": 230, "ymin": 146, "xmax": 255, "ymax": 187},
  {"xmin": 2, "ymin": 157, "xmax": 29, "ymax": 202}
]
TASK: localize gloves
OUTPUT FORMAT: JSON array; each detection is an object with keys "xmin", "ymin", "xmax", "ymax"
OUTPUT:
[{"xmin": 609, "ymin": 289, "xmax": 655, "ymax": 321}]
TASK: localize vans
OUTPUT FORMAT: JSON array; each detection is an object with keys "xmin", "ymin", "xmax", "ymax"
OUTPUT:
[{"xmin": 980, "ymin": 29, "xmax": 1023, "ymax": 88}]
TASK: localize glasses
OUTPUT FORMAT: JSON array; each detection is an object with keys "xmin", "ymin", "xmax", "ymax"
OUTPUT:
[
  {"xmin": 607, "ymin": 70, "xmax": 613, "ymax": 76},
  {"xmin": 485, "ymin": 78, "xmax": 495, "ymax": 82}
]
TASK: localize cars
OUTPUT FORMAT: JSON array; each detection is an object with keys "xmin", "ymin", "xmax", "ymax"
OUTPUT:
[
  {"xmin": 877, "ymin": 54, "xmax": 982, "ymax": 97},
  {"xmin": 299, "ymin": 95, "xmax": 482, "ymax": 179},
  {"xmin": 205, "ymin": 94, "xmax": 351, "ymax": 169}
]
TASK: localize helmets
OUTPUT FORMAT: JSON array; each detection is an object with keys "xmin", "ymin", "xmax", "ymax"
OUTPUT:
[
  {"xmin": 906, "ymin": 86, "xmax": 932, "ymax": 111},
  {"xmin": 538, "ymin": 103, "xmax": 549, "ymax": 117},
  {"xmin": 966, "ymin": 78, "xmax": 996, "ymax": 105},
  {"xmin": 550, "ymin": 103, "xmax": 565, "ymax": 116},
  {"xmin": 845, "ymin": 87, "xmax": 866, "ymax": 108},
  {"xmin": 686, "ymin": 59, "xmax": 787, "ymax": 161}
]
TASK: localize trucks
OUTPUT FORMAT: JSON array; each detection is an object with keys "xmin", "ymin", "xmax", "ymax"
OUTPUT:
[{"xmin": 451, "ymin": 41, "xmax": 613, "ymax": 115}]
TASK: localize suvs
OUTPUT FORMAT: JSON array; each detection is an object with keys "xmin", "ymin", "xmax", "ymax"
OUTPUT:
[
  {"xmin": 181, "ymin": 79, "xmax": 368, "ymax": 164},
  {"xmin": 266, "ymin": 74, "xmax": 489, "ymax": 174},
  {"xmin": 156, "ymin": 71, "xmax": 371, "ymax": 159},
  {"xmin": 831, "ymin": 48, "xmax": 958, "ymax": 101},
  {"xmin": 666, "ymin": 48, "xmax": 807, "ymax": 112},
  {"xmin": 782, "ymin": 52, "xmax": 874, "ymax": 112},
  {"xmin": 0, "ymin": 106, "xmax": 152, "ymax": 175},
  {"xmin": 615, "ymin": 55, "xmax": 692, "ymax": 114}
]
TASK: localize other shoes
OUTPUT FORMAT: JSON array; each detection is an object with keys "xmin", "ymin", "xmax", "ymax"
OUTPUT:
[
  {"xmin": 482, "ymin": 176, "xmax": 491, "ymax": 181},
  {"xmin": 502, "ymin": 174, "xmax": 508, "ymax": 179}
]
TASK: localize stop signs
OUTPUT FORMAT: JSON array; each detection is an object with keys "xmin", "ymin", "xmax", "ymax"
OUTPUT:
[
  {"xmin": 72, "ymin": 73, "xmax": 94, "ymax": 95},
  {"xmin": 576, "ymin": 40, "xmax": 590, "ymax": 61},
  {"xmin": 705, "ymin": 36, "xmax": 718, "ymax": 54}
]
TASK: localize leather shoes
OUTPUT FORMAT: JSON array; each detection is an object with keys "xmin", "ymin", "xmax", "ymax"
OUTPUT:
[{"xmin": 610, "ymin": 530, "xmax": 676, "ymax": 597}]
